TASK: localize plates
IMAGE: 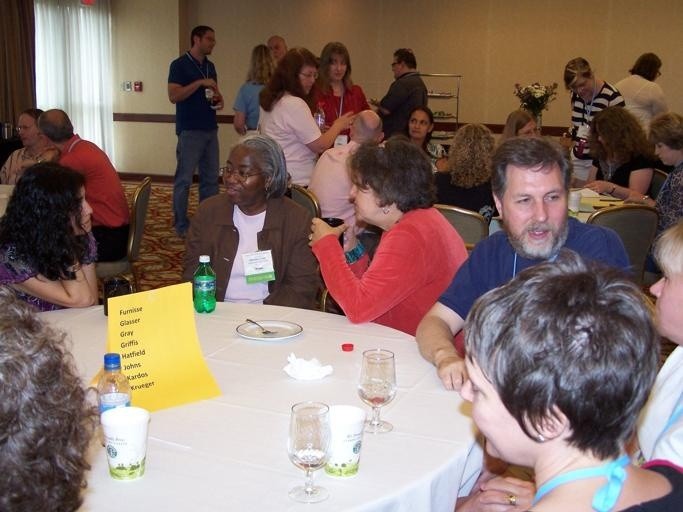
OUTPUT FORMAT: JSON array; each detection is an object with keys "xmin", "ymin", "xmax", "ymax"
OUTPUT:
[{"xmin": 236, "ymin": 320, "xmax": 304, "ymax": 341}]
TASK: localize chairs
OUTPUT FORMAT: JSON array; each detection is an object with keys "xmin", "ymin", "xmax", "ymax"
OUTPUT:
[
  {"xmin": 646, "ymin": 168, "xmax": 668, "ymax": 201},
  {"xmin": 94, "ymin": 176, "xmax": 152, "ymax": 294},
  {"xmin": 432, "ymin": 204, "xmax": 489, "ymax": 258},
  {"xmin": 284, "ymin": 183, "xmax": 328, "ymax": 312},
  {"xmin": 586, "ymin": 203, "xmax": 660, "ymax": 294}
]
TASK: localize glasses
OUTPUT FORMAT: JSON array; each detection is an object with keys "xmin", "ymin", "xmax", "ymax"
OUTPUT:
[
  {"xmin": 216, "ymin": 166, "xmax": 264, "ymax": 184},
  {"xmin": 299, "ymin": 70, "xmax": 320, "ymax": 80}
]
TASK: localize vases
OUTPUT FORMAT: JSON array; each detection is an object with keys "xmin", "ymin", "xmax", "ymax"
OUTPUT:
[{"xmin": 528, "ymin": 110, "xmax": 542, "ymax": 136}]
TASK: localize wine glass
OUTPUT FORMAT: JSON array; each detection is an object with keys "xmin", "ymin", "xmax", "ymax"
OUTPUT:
[
  {"xmin": 288, "ymin": 401, "xmax": 330, "ymax": 503},
  {"xmin": 358, "ymin": 349, "xmax": 397, "ymax": 437}
]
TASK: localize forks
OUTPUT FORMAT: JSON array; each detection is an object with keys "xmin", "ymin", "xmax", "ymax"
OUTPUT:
[{"xmin": 246, "ymin": 318, "xmax": 279, "ymax": 334}]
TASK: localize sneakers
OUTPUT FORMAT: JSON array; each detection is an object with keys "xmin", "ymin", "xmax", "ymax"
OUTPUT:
[{"xmin": 177, "ymin": 229, "xmax": 188, "ymax": 239}]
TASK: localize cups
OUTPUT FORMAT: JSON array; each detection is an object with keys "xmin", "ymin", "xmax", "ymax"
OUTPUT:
[
  {"xmin": 101, "ymin": 405, "xmax": 151, "ymax": 482},
  {"xmin": 327, "ymin": 405, "xmax": 365, "ymax": 477},
  {"xmin": 317, "ymin": 217, "xmax": 345, "ymax": 252},
  {"xmin": 567, "ymin": 192, "xmax": 581, "ymax": 212}
]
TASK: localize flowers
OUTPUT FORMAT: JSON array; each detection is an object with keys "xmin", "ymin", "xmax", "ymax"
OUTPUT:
[{"xmin": 512, "ymin": 82, "xmax": 558, "ymax": 115}]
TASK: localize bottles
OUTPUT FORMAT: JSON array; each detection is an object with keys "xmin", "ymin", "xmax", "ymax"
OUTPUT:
[
  {"xmin": 314, "ymin": 102, "xmax": 326, "ymax": 132},
  {"xmin": 96, "ymin": 354, "xmax": 133, "ymax": 446},
  {"xmin": 192, "ymin": 254, "xmax": 219, "ymax": 313}
]
